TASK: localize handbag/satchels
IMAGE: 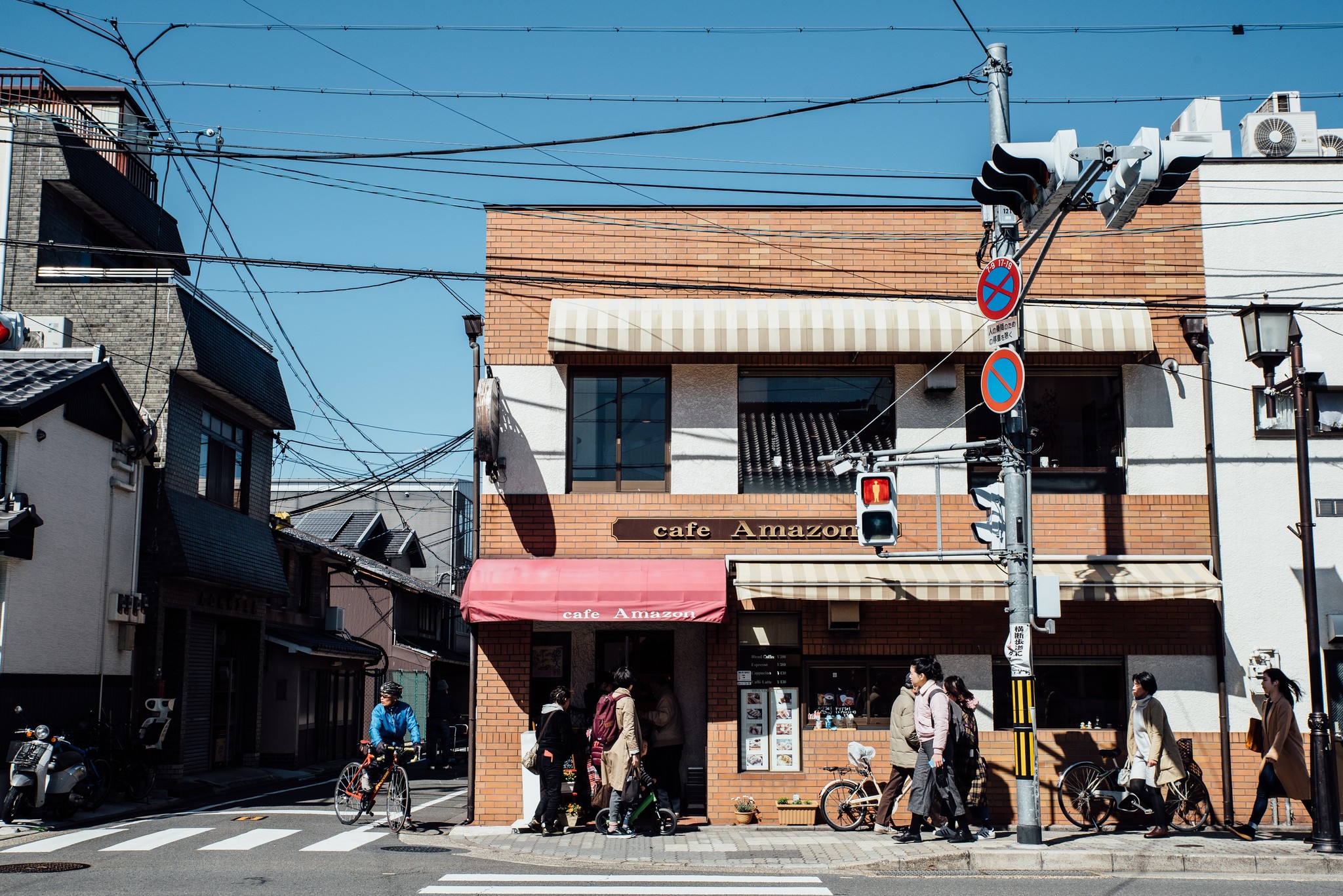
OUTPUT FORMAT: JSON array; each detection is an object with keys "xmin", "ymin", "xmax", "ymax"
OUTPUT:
[
  {"xmin": 1245, "ymin": 700, "xmax": 1267, "ymax": 753},
  {"xmin": 1117, "ymin": 758, "xmax": 1132, "ymax": 788},
  {"xmin": 621, "ymin": 763, "xmax": 642, "ymax": 803},
  {"xmin": 520, "ymin": 743, "xmax": 540, "ymax": 775}
]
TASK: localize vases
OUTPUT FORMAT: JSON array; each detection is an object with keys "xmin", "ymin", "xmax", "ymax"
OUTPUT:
[
  {"xmin": 561, "ymin": 781, "xmax": 576, "ymax": 793},
  {"xmin": 557, "ymin": 813, "xmax": 580, "ymax": 826},
  {"xmin": 734, "ymin": 810, "xmax": 754, "ymax": 825}
]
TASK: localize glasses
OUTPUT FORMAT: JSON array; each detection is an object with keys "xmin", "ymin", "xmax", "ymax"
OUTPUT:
[{"xmin": 379, "ymin": 694, "xmax": 394, "ymax": 698}]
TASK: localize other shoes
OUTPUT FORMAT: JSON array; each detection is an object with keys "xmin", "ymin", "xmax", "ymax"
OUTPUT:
[
  {"xmin": 1303, "ymin": 831, "xmax": 1313, "ymax": 844},
  {"xmin": 667, "ymin": 808, "xmax": 673, "ymax": 811},
  {"xmin": 675, "ymin": 813, "xmax": 680, "ymax": 818},
  {"xmin": 873, "ymin": 822, "xmax": 993, "ymax": 843},
  {"xmin": 1227, "ymin": 820, "xmax": 1258, "ymax": 841}
]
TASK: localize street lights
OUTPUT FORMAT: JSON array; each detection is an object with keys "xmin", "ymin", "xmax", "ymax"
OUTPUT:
[{"xmin": 1232, "ymin": 289, "xmax": 1343, "ymax": 853}]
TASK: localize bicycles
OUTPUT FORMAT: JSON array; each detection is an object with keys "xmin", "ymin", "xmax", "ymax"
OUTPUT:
[
  {"xmin": 76, "ymin": 732, "xmax": 157, "ymax": 812},
  {"xmin": 334, "ymin": 740, "xmax": 422, "ymax": 832},
  {"xmin": 595, "ymin": 780, "xmax": 677, "ymax": 836},
  {"xmin": 1056, "ymin": 738, "xmax": 1210, "ymax": 832},
  {"xmin": 818, "ymin": 741, "xmax": 959, "ymax": 831}
]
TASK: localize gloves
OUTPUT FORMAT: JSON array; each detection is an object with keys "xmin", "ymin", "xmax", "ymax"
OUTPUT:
[
  {"xmin": 376, "ymin": 741, "xmax": 390, "ymax": 756},
  {"xmin": 413, "ymin": 742, "xmax": 424, "ymax": 758}
]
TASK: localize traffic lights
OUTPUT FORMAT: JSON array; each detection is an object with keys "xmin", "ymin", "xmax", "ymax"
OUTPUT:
[
  {"xmin": 855, "ymin": 472, "xmax": 897, "ymax": 546},
  {"xmin": 971, "ymin": 129, "xmax": 1078, "ymax": 230},
  {"xmin": 970, "ymin": 482, "xmax": 1005, "ymax": 550},
  {"xmin": 1098, "ymin": 127, "xmax": 1214, "ymax": 230}
]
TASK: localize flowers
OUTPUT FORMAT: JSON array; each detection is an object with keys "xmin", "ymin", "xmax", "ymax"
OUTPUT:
[
  {"xmin": 557, "ymin": 801, "xmax": 585, "ymax": 815},
  {"xmin": 560, "ymin": 769, "xmax": 577, "ymax": 782},
  {"xmin": 731, "ymin": 795, "xmax": 758, "ymax": 812}
]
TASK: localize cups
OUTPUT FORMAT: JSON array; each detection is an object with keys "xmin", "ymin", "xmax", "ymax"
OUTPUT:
[
  {"xmin": 787, "ymin": 740, "xmax": 791, "ymax": 743},
  {"xmin": 816, "ymin": 717, "xmax": 854, "ymax": 729}
]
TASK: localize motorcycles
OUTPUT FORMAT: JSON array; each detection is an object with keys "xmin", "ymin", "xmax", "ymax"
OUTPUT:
[{"xmin": 2, "ymin": 706, "xmax": 107, "ymax": 825}]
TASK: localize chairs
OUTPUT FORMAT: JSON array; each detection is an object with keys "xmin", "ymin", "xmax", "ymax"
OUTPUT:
[{"xmin": 436, "ymin": 724, "xmax": 468, "ymax": 757}]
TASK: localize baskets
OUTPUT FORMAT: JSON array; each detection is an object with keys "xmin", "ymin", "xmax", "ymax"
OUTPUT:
[{"xmin": 1176, "ymin": 738, "xmax": 1194, "ymax": 763}]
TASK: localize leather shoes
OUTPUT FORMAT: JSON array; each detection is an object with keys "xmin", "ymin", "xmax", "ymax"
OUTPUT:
[{"xmin": 1144, "ymin": 825, "xmax": 1171, "ymax": 839}]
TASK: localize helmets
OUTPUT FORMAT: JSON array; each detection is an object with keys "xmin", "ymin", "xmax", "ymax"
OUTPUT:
[{"xmin": 380, "ymin": 681, "xmax": 404, "ymax": 695}]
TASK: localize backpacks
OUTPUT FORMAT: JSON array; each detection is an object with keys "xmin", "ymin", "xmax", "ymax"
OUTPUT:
[{"xmin": 592, "ymin": 692, "xmax": 633, "ymax": 746}]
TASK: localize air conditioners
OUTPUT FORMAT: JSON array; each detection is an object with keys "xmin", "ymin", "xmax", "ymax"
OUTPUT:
[{"xmin": 1163, "ymin": 91, "xmax": 1342, "ymax": 160}]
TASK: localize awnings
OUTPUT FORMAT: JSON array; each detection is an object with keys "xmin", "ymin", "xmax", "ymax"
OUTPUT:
[
  {"xmin": 732, "ymin": 553, "xmax": 1223, "ymax": 605},
  {"xmin": 461, "ymin": 557, "xmax": 730, "ymax": 625}
]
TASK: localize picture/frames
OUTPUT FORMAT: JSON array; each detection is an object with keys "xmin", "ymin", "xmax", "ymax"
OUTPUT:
[{"xmin": 768, "ymin": 686, "xmax": 800, "ymax": 772}]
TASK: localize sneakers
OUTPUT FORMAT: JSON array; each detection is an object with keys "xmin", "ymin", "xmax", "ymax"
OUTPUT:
[
  {"xmin": 402, "ymin": 818, "xmax": 414, "ymax": 830},
  {"xmin": 630, "ymin": 826, "xmax": 642, "ymax": 835},
  {"xmin": 620, "ymin": 826, "xmax": 637, "ymax": 837},
  {"xmin": 443, "ymin": 765, "xmax": 452, "ymax": 770},
  {"xmin": 542, "ymin": 826, "xmax": 565, "ymax": 837},
  {"xmin": 360, "ymin": 774, "xmax": 374, "ymax": 792},
  {"xmin": 529, "ymin": 817, "xmax": 544, "ymax": 834},
  {"xmin": 429, "ymin": 766, "xmax": 435, "ymax": 770},
  {"xmin": 606, "ymin": 827, "xmax": 632, "ymax": 839}
]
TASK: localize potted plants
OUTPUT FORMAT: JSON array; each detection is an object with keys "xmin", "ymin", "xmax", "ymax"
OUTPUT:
[{"xmin": 775, "ymin": 796, "xmax": 819, "ymax": 826}]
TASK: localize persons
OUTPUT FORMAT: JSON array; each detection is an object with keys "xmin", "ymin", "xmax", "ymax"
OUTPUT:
[
  {"xmin": 526, "ymin": 686, "xmax": 576, "ymax": 837},
  {"xmin": 360, "ymin": 680, "xmax": 425, "ymax": 831},
  {"xmin": 599, "ymin": 666, "xmax": 641, "ymax": 839},
  {"xmin": 1227, "ymin": 667, "xmax": 1319, "ymax": 843},
  {"xmin": 873, "ymin": 672, "xmax": 963, "ymax": 835},
  {"xmin": 848, "ymin": 673, "xmax": 891, "ymax": 718},
  {"xmin": 890, "ymin": 656, "xmax": 976, "ymax": 844},
  {"xmin": 637, "ymin": 673, "xmax": 685, "ymax": 819},
  {"xmin": 620, "ymin": 736, "xmax": 657, "ymax": 837},
  {"xmin": 584, "ymin": 671, "xmax": 613, "ymax": 733},
  {"xmin": 940, "ymin": 676, "xmax": 997, "ymax": 839},
  {"xmin": 1125, "ymin": 671, "xmax": 1187, "ymax": 839}
]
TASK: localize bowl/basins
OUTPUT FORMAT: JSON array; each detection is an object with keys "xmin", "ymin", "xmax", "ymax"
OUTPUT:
[
  {"xmin": 843, "ymin": 697, "xmax": 854, "ymax": 705},
  {"xmin": 824, "ymin": 693, "xmax": 834, "ymax": 703}
]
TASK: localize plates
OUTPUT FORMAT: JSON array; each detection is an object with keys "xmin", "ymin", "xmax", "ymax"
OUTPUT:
[
  {"xmin": 785, "ymin": 741, "xmax": 792, "ymax": 744},
  {"xmin": 776, "ymin": 711, "xmax": 789, "ymax": 719},
  {"xmin": 747, "ymin": 755, "xmax": 762, "ymax": 766},
  {"xmin": 779, "ymin": 699, "xmax": 792, "ymax": 704},
  {"xmin": 748, "ymin": 709, "xmax": 760, "ymax": 719},
  {"xmin": 751, "ymin": 742, "xmax": 760, "ymax": 747},
  {"xmin": 777, "ymin": 755, "xmax": 792, "ymax": 766},
  {"xmin": 777, "ymin": 744, "xmax": 781, "ymax": 750}
]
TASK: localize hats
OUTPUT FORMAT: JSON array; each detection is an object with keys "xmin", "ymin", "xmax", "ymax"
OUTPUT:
[
  {"xmin": 436, "ymin": 680, "xmax": 448, "ymax": 692},
  {"xmin": 905, "ymin": 672, "xmax": 913, "ymax": 684}
]
